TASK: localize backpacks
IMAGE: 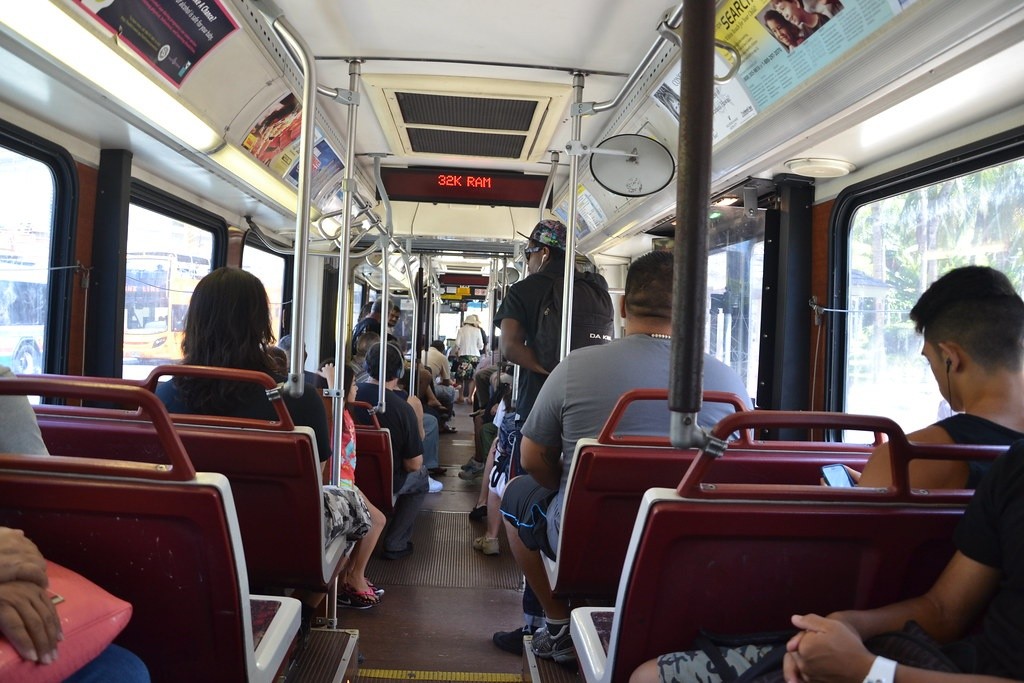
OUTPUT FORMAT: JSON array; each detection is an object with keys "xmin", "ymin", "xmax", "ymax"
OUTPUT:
[
  {"xmin": 700, "ymin": 618, "xmax": 982, "ymax": 683},
  {"xmin": 532, "ymin": 271, "xmax": 616, "ymax": 371}
]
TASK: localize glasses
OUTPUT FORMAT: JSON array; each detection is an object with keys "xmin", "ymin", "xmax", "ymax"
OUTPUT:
[{"xmin": 525, "ymin": 247, "xmax": 541, "ymax": 260}]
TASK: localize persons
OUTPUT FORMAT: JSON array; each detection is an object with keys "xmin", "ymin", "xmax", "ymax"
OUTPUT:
[
  {"xmin": 630, "ymin": 438, "xmax": 1024, "ymax": 683},
  {"xmin": 150, "ymin": 266, "xmax": 372, "ymax": 539},
  {"xmin": 0, "ymin": 364, "xmax": 50, "ymax": 456},
  {"xmin": 449, "ymin": 219, "xmax": 613, "ymax": 554},
  {"xmin": 492, "ymin": 252, "xmax": 753, "ymax": 666},
  {"xmin": 818, "ymin": 266, "xmax": 1024, "ymax": 490},
  {"xmin": 0, "ymin": 528, "xmax": 150, "ymax": 683},
  {"xmin": 259, "ymin": 294, "xmax": 456, "ymax": 607}
]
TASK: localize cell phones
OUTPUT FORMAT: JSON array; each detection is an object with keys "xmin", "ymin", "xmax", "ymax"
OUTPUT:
[{"xmin": 821, "ymin": 464, "xmax": 855, "ymax": 488}]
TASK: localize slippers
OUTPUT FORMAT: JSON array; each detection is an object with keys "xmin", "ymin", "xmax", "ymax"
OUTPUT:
[{"xmin": 339, "ymin": 575, "xmax": 385, "ymax": 604}]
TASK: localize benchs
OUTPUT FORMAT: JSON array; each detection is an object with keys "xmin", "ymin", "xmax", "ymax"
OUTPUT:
[
  {"xmin": 541, "ymin": 388, "xmax": 1012, "ymax": 683},
  {"xmin": 0, "ymin": 366, "xmax": 393, "ymax": 683}
]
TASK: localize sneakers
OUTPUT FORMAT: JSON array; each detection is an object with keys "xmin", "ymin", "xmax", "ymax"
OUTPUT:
[
  {"xmin": 493, "ymin": 624, "xmax": 542, "ymax": 655},
  {"xmin": 530, "ymin": 624, "xmax": 576, "ymax": 661},
  {"xmin": 427, "ymin": 475, "xmax": 443, "ymax": 493}
]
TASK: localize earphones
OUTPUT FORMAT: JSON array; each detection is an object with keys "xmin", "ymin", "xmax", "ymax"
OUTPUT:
[
  {"xmin": 541, "ymin": 254, "xmax": 546, "ymax": 263},
  {"xmin": 946, "ymin": 358, "xmax": 951, "ymax": 373}
]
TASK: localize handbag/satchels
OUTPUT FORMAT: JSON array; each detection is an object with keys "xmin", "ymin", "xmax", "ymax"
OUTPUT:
[{"xmin": 0, "ymin": 559, "xmax": 133, "ymax": 683}]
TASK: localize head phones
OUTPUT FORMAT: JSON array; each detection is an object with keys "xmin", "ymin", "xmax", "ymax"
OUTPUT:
[{"xmin": 362, "ymin": 342, "xmax": 405, "ymax": 379}]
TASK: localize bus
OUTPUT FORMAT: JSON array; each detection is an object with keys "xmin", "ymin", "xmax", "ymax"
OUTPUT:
[
  {"xmin": 123, "ymin": 250, "xmax": 265, "ymax": 364},
  {"xmin": 0, "ymin": 232, "xmax": 49, "ymax": 374}
]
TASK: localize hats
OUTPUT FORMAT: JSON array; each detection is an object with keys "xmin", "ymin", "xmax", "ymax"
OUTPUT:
[
  {"xmin": 464, "ymin": 315, "xmax": 481, "ymax": 324},
  {"xmin": 516, "ymin": 219, "xmax": 567, "ymax": 249}
]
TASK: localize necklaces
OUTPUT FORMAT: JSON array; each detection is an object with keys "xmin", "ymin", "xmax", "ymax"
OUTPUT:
[{"xmin": 624, "ymin": 331, "xmax": 674, "ymax": 340}]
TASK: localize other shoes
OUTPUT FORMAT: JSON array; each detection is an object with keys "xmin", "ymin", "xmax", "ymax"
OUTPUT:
[
  {"xmin": 458, "ymin": 457, "xmax": 483, "ymax": 480},
  {"xmin": 383, "ymin": 541, "xmax": 414, "ymax": 560},
  {"xmin": 468, "ymin": 409, "xmax": 481, "ymax": 416},
  {"xmin": 428, "ymin": 467, "xmax": 447, "ymax": 475},
  {"xmin": 473, "ymin": 535, "xmax": 500, "ymax": 553},
  {"xmin": 439, "ymin": 424, "xmax": 458, "ymax": 434},
  {"xmin": 469, "ymin": 504, "xmax": 487, "ymax": 516}
]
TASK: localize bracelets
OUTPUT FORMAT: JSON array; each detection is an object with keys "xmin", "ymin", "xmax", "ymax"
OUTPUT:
[{"xmin": 862, "ymin": 656, "xmax": 897, "ymax": 683}]
